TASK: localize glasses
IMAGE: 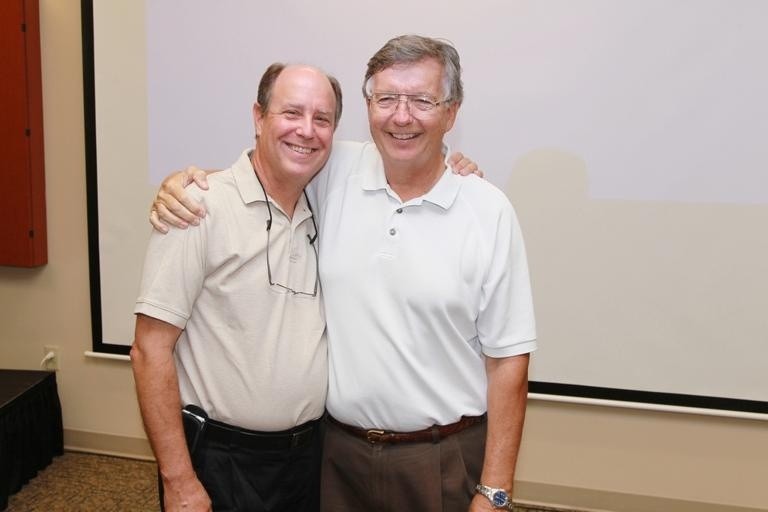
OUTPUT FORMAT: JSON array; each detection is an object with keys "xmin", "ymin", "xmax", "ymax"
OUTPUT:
[
  {"xmin": 267, "ymin": 222, "xmax": 319, "ymax": 299},
  {"xmin": 369, "ymin": 91, "xmax": 451, "ymax": 120}
]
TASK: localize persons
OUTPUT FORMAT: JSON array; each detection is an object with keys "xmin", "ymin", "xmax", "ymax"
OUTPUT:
[
  {"xmin": 144, "ymin": 35, "xmax": 542, "ymax": 512},
  {"xmin": 127, "ymin": 60, "xmax": 486, "ymax": 512}
]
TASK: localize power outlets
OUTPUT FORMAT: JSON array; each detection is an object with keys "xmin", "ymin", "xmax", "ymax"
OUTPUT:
[{"xmin": 42, "ymin": 344, "xmax": 62, "ymax": 370}]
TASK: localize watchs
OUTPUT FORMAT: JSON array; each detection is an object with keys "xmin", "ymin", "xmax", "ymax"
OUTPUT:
[{"xmin": 473, "ymin": 480, "xmax": 516, "ymax": 512}]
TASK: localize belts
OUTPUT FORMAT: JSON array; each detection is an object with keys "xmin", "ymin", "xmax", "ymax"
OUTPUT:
[
  {"xmin": 324, "ymin": 414, "xmax": 489, "ymax": 446},
  {"xmin": 202, "ymin": 421, "xmax": 316, "ymax": 450}
]
